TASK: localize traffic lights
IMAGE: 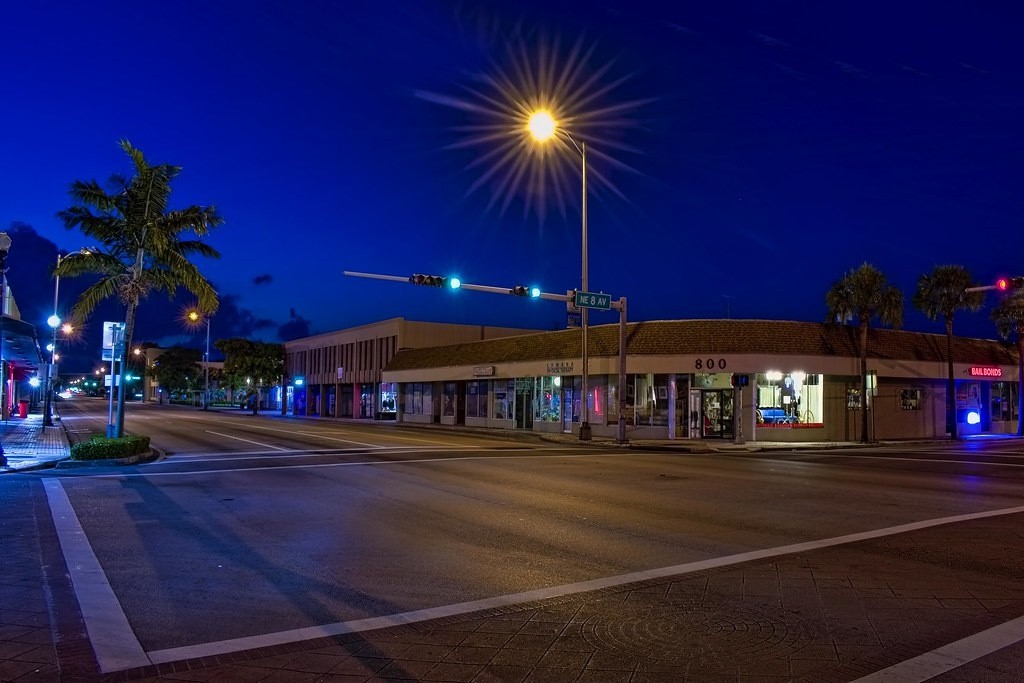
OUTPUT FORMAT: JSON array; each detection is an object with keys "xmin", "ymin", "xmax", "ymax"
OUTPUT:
[
  {"xmin": 410, "ymin": 274, "xmax": 460, "ymax": 289},
  {"xmin": 512, "ymin": 285, "xmax": 541, "ymax": 298},
  {"xmin": 996, "ymin": 276, "xmax": 1024, "ymax": 291}
]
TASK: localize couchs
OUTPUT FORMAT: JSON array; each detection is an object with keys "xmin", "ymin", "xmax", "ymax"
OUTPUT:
[{"xmin": 756, "ymin": 409, "xmax": 797, "ymax": 424}]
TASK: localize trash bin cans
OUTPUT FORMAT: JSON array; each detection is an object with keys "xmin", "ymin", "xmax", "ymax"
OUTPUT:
[{"xmin": 19, "ymin": 400, "xmax": 29, "ymax": 418}]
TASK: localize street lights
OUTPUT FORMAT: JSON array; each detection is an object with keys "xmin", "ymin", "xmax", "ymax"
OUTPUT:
[
  {"xmin": 135, "ymin": 348, "xmax": 149, "ymax": 401},
  {"xmin": 527, "ymin": 109, "xmax": 593, "ymax": 440},
  {"xmin": 189, "ymin": 311, "xmax": 210, "ymax": 395},
  {"xmin": 50, "ymin": 249, "xmax": 91, "ymax": 416}
]
